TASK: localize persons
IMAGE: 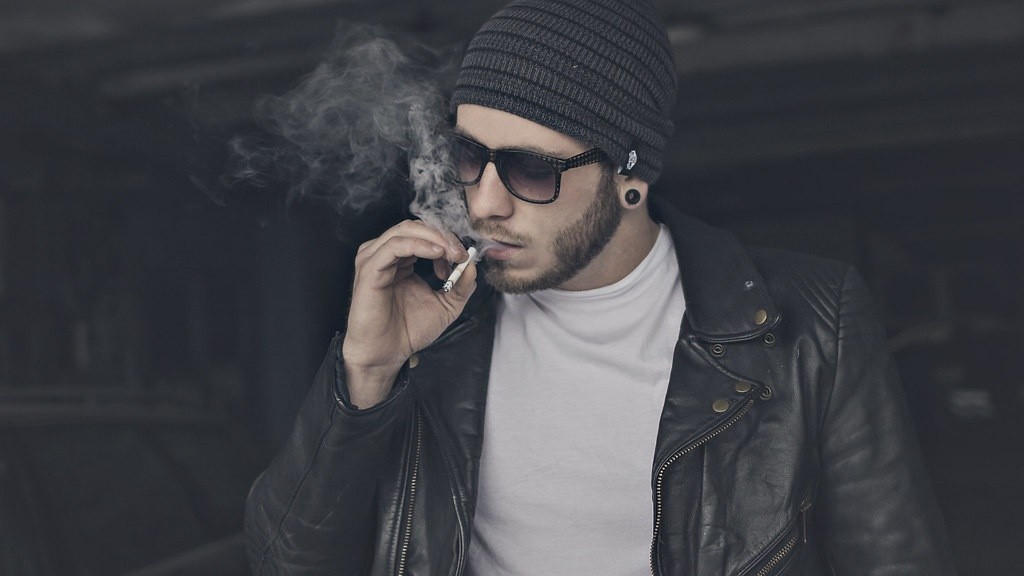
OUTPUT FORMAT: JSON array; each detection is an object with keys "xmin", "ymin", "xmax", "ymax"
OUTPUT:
[{"xmin": 242, "ymin": 0, "xmax": 955, "ymax": 576}]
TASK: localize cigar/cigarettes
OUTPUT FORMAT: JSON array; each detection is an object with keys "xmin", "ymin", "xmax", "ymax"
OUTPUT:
[{"xmin": 442, "ymin": 246, "xmax": 476, "ymax": 294}]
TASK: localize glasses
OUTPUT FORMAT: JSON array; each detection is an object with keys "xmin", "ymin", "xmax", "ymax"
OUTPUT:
[{"xmin": 434, "ymin": 121, "xmax": 609, "ymax": 204}]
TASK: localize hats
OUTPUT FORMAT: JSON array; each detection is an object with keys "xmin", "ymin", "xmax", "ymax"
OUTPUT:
[{"xmin": 449, "ymin": 0, "xmax": 677, "ymax": 186}]
